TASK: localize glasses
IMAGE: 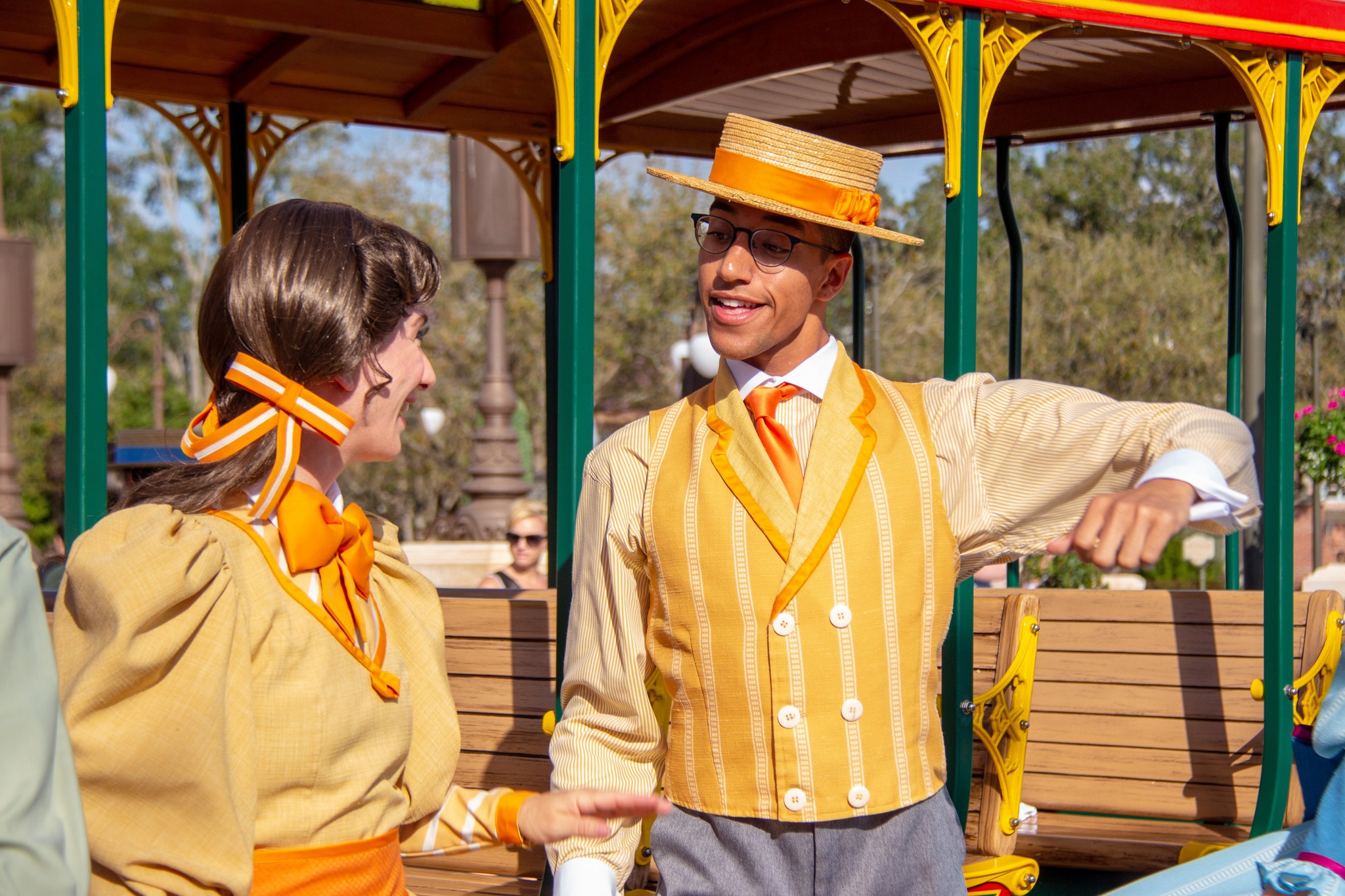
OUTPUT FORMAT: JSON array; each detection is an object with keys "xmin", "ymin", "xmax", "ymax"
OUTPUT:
[
  {"xmin": 689, "ymin": 209, "xmax": 847, "ymax": 268},
  {"xmin": 507, "ymin": 531, "xmax": 549, "ymax": 547}
]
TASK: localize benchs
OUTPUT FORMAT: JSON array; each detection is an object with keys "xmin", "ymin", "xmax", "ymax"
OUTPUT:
[
  {"xmin": 390, "ymin": 593, "xmax": 1047, "ymax": 896},
  {"xmin": 973, "ymin": 587, "xmax": 1345, "ymax": 871}
]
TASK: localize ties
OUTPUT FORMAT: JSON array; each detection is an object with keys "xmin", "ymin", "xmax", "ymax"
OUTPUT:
[{"xmin": 743, "ymin": 381, "xmax": 804, "ymax": 510}]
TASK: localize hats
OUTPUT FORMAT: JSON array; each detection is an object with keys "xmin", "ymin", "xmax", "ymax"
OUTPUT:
[{"xmin": 645, "ymin": 111, "xmax": 928, "ymax": 247}]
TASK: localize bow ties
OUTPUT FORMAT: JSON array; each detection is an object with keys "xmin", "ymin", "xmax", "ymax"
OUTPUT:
[{"xmin": 275, "ymin": 478, "xmax": 374, "ymax": 650}]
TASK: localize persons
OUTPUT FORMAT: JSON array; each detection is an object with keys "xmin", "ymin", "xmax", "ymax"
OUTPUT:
[
  {"xmin": 50, "ymin": 200, "xmax": 676, "ymax": 896},
  {"xmin": 476, "ymin": 499, "xmax": 550, "ymax": 590},
  {"xmin": 543, "ymin": 112, "xmax": 1265, "ymax": 896},
  {"xmin": 0, "ymin": 506, "xmax": 90, "ymax": 896}
]
